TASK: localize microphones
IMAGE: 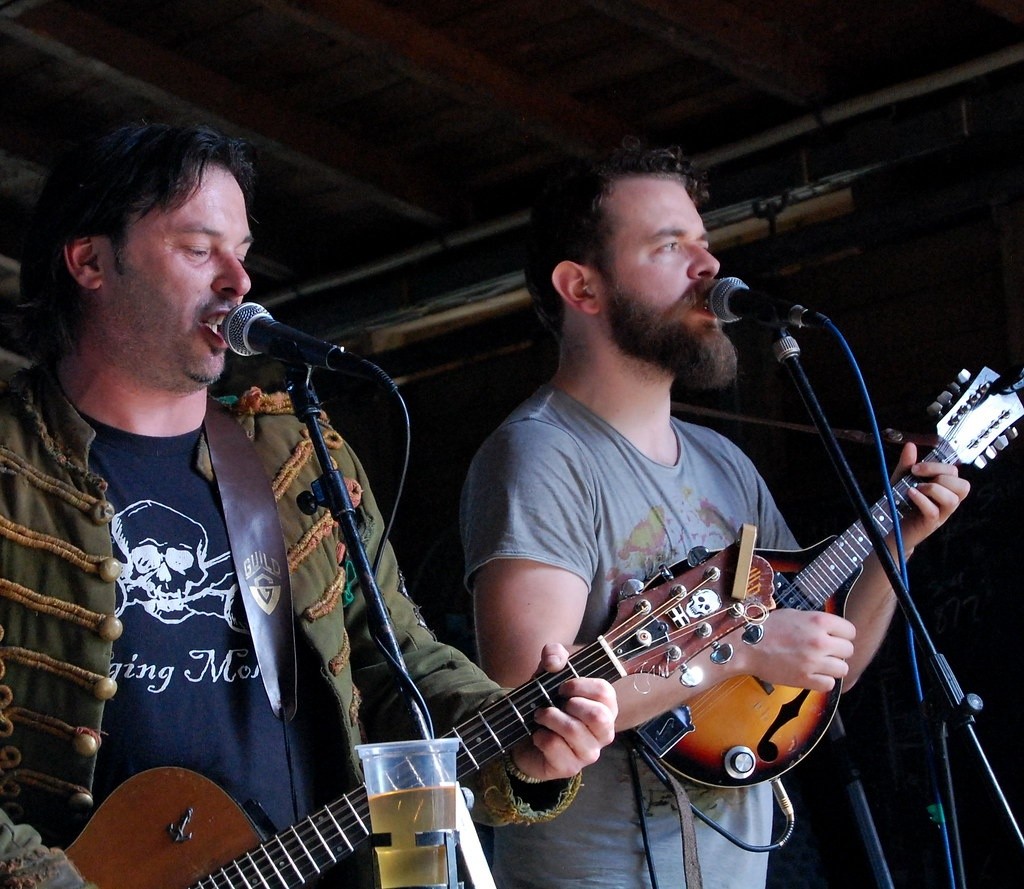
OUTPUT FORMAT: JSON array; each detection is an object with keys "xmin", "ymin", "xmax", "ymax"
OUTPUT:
[
  {"xmin": 709, "ymin": 278, "xmax": 830, "ymax": 330},
  {"xmin": 222, "ymin": 301, "xmax": 399, "ymax": 394}
]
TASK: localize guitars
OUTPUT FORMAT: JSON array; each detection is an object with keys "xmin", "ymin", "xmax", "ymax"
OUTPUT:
[
  {"xmin": 609, "ymin": 364, "xmax": 1023, "ymax": 789},
  {"xmin": 65, "ymin": 525, "xmax": 773, "ymax": 889}
]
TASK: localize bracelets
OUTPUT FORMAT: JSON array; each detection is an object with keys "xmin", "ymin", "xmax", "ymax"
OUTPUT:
[{"xmin": 506, "ymin": 753, "xmax": 545, "ymax": 784}]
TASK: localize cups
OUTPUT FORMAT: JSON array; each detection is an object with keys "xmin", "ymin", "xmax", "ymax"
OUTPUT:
[{"xmin": 354, "ymin": 737, "xmax": 461, "ymax": 889}]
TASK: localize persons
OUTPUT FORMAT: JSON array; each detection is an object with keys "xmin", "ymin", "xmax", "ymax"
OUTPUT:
[
  {"xmin": 459, "ymin": 142, "xmax": 970, "ymax": 889},
  {"xmin": 1, "ymin": 120, "xmax": 620, "ymax": 888}
]
